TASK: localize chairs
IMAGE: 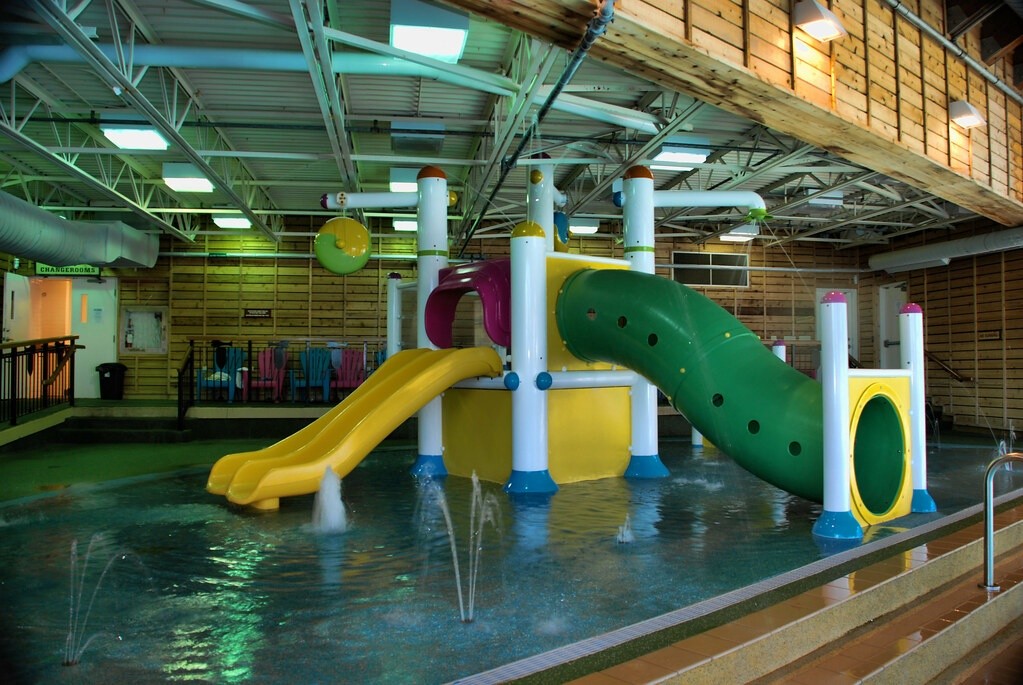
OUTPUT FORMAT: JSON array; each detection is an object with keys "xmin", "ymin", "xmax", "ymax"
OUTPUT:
[
  {"xmin": 330, "ymin": 349, "xmax": 363, "ymax": 403},
  {"xmin": 243, "ymin": 347, "xmax": 290, "ymax": 403},
  {"xmin": 288, "ymin": 346, "xmax": 335, "ymax": 403},
  {"xmin": 196, "ymin": 346, "xmax": 246, "ymax": 404}
]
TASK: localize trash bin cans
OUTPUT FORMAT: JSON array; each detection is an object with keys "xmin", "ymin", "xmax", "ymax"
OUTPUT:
[{"xmin": 95, "ymin": 362, "xmax": 128, "ymax": 401}]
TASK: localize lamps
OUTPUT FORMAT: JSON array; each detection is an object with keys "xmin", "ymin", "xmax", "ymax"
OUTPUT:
[
  {"xmin": 950, "ymin": 99, "xmax": 987, "ymax": 130},
  {"xmin": 392, "ymin": 217, "xmax": 418, "ymax": 231},
  {"xmin": 390, "ymin": 167, "xmax": 421, "ymax": 193},
  {"xmin": 796, "ymin": 189, "xmax": 844, "ymax": 205},
  {"xmin": 99, "ymin": 112, "xmax": 169, "ymax": 151},
  {"xmin": 796, "ymin": 0, "xmax": 847, "ymax": 42},
  {"xmin": 389, "ymin": 0, "xmax": 470, "ymax": 64},
  {"xmin": 391, "ymin": 121, "xmax": 446, "ymax": 155},
  {"xmin": 647, "ymin": 136, "xmax": 711, "ymax": 171},
  {"xmin": 162, "ymin": 162, "xmax": 215, "ymax": 192},
  {"xmin": 569, "ymin": 218, "xmax": 600, "ymax": 234},
  {"xmin": 212, "ymin": 214, "xmax": 253, "ymax": 229},
  {"xmin": 720, "ymin": 225, "xmax": 760, "ymax": 242}
]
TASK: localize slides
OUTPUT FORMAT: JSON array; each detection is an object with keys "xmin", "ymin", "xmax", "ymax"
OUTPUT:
[
  {"xmin": 559, "ymin": 269, "xmax": 914, "ymax": 530},
  {"xmin": 205, "ymin": 346, "xmax": 504, "ymax": 506}
]
